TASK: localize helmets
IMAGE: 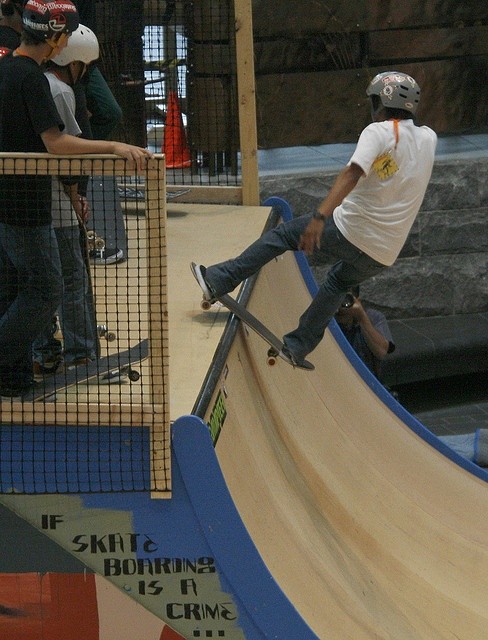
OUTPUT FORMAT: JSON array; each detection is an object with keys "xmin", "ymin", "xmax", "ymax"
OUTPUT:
[
  {"xmin": 24, "ymin": 0, "xmax": 80, "ymax": 40},
  {"xmin": 51, "ymin": 23, "xmax": 101, "ymax": 67},
  {"xmin": 366, "ymin": 71, "xmax": 422, "ymax": 122}
]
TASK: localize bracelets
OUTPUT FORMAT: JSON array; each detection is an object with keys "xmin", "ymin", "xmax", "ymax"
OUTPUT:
[{"xmin": 310, "ymin": 207, "xmax": 328, "ymax": 222}]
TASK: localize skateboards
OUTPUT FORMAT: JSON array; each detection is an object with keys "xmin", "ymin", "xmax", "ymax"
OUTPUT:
[
  {"xmin": 85, "ymin": 176, "xmax": 127, "ymax": 264},
  {"xmin": 19, "ymin": 338, "xmax": 149, "ymax": 403},
  {"xmin": 190, "ymin": 262, "xmax": 315, "ymax": 371},
  {"xmin": 119, "ymin": 186, "xmax": 191, "ymax": 200},
  {"xmin": 76, "ymin": 214, "xmax": 116, "ymax": 361}
]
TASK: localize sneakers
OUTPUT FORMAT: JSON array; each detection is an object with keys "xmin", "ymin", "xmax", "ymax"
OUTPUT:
[
  {"xmin": 284, "ymin": 340, "xmax": 298, "ymax": 368},
  {"xmin": 88, "ymin": 246, "xmax": 124, "ymax": 265},
  {"xmin": 1, "ymin": 381, "xmax": 57, "ymax": 405},
  {"xmin": 194, "ymin": 265, "xmax": 214, "ymax": 303},
  {"xmin": 63, "ymin": 357, "xmax": 131, "ymax": 386},
  {"xmin": 33, "ymin": 359, "xmax": 63, "ymax": 377}
]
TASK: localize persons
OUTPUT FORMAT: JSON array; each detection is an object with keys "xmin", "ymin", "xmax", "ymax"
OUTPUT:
[
  {"xmin": 87, "ymin": 246, "xmax": 126, "ymax": 266},
  {"xmin": 0, "ymin": 1, "xmax": 30, "ymax": 61},
  {"xmin": 0, "ymin": 0, "xmax": 156, "ymax": 403},
  {"xmin": 193, "ymin": 68, "xmax": 440, "ymax": 370},
  {"xmin": 30, "ymin": 21, "xmax": 128, "ymax": 386},
  {"xmin": 83, "ymin": 63, "xmax": 124, "ymax": 142},
  {"xmin": 332, "ymin": 282, "xmax": 397, "ymax": 384}
]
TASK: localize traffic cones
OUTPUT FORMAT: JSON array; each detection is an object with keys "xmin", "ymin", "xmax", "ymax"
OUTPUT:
[{"xmin": 159, "ymin": 67, "xmax": 201, "ymax": 169}]
